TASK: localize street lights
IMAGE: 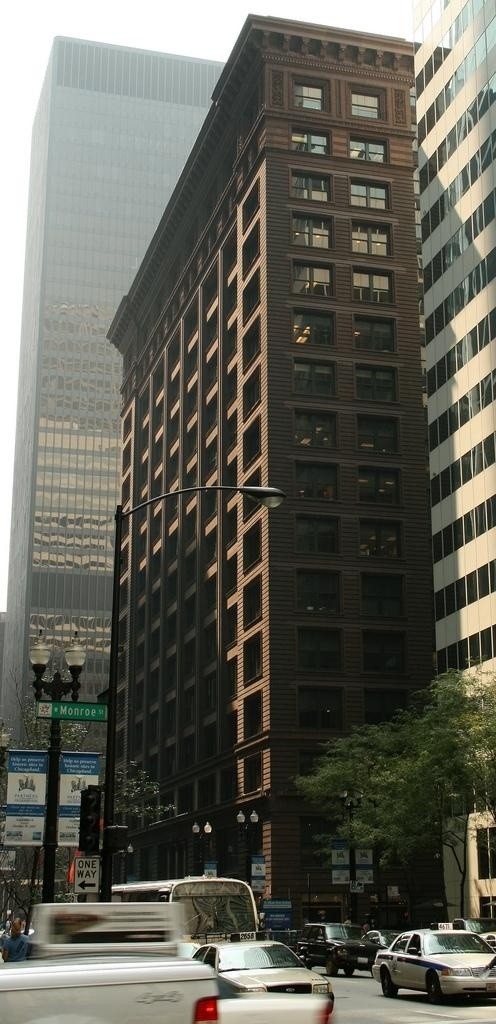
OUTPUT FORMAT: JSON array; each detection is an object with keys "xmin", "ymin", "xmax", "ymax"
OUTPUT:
[
  {"xmin": 192, "ymin": 821, "xmax": 212, "ymax": 875},
  {"xmin": 28, "ymin": 627, "xmax": 87, "ymax": 902},
  {"xmin": 97, "ymin": 486, "xmax": 286, "ymax": 903},
  {"xmin": 117, "ymin": 844, "xmax": 133, "ymax": 884},
  {"xmin": 236, "ymin": 810, "xmax": 260, "ymax": 884}
]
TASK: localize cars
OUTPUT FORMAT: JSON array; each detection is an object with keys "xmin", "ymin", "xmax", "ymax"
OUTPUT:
[{"xmin": 362, "ymin": 918, "xmax": 495, "ymax": 1003}]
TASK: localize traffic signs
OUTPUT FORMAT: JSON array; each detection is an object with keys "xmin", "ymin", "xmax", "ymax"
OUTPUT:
[{"xmin": 74, "ymin": 857, "xmax": 101, "ymax": 894}]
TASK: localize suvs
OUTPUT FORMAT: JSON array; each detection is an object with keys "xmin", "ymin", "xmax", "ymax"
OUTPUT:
[{"xmin": 299, "ymin": 920, "xmax": 385, "ymax": 975}]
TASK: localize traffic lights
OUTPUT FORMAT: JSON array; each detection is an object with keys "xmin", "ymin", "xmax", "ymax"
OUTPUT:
[{"xmin": 78, "ymin": 789, "xmax": 102, "ymax": 852}]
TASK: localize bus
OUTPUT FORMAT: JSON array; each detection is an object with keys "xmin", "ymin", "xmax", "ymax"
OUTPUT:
[{"xmin": 113, "ymin": 878, "xmax": 257, "ymax": 943}]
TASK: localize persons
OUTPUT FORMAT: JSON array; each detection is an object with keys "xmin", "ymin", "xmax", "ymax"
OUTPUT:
[
  {"xmin": 343, "ymin": 915, "xmax": 352, "ymax": 925},
  {"xmin": 0, "ymin": 918, "xmax": 30, "ymax": 962}
]
